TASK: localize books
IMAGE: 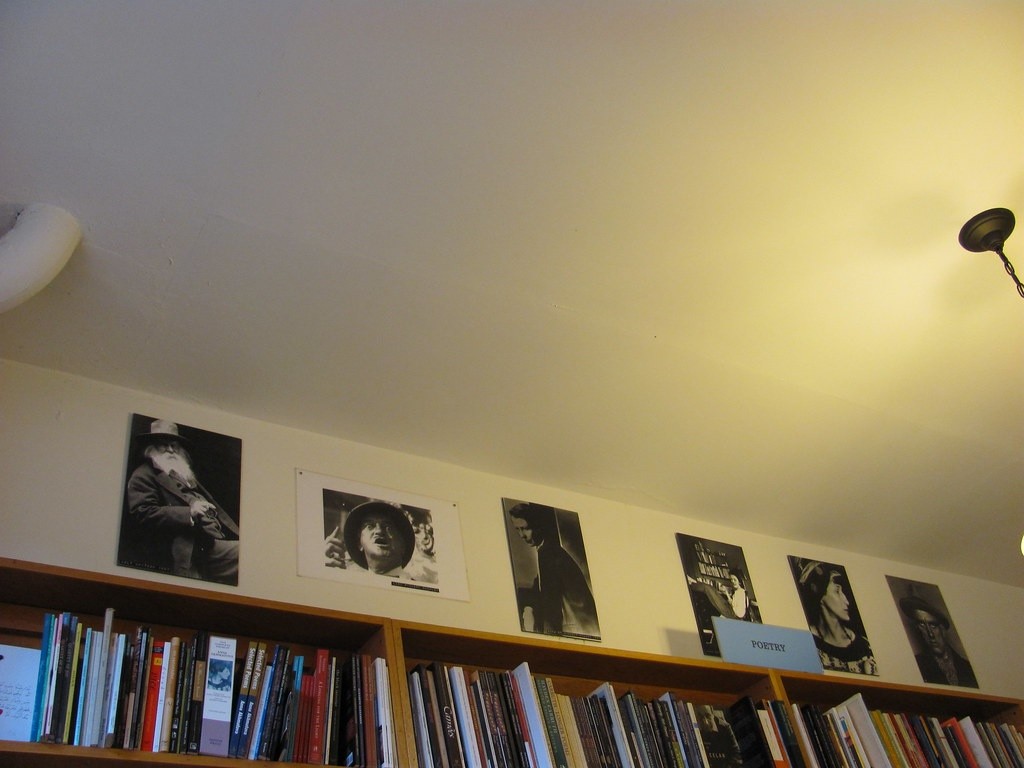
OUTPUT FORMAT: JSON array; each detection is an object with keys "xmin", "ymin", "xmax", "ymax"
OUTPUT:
[
  {"xmin": 0, "ymin": 606, "xmax": 402, "ymax": 768},
  {"xmin": 406, "ymin": 661, "xmax": 809, "ymax": 768},
  {"xmin": 791, "ymin": 691, "xmax": 1023, "ymax": 768}
]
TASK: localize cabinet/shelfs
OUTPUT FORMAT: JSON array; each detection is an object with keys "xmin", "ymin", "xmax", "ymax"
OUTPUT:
[{"xmin": 0, "ymin": 551, "xmax": 1024, "ymax": 768}]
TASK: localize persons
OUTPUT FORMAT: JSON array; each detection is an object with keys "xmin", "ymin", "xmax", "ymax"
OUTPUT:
[
  {"xmin": 900, "ymin": 583, "xmax": 980, "ymax": 688},
  {"xmin": 509, "ymin": 502, "xmax": 602, "ymax": 641},
  {"xmin": 127, "ymin": 419, "xmax": 241, "ymax": 587},
  {"xmin": 324, "ymin": 500, "xmax": 417, "ymax": 581},
  {"xmin": 686, "ymin": 567, "xmax": 751, "ymax": 620},
  {"xmin": 800, "ymin": 560, "xmax": 880, "ymax": 675}
]
